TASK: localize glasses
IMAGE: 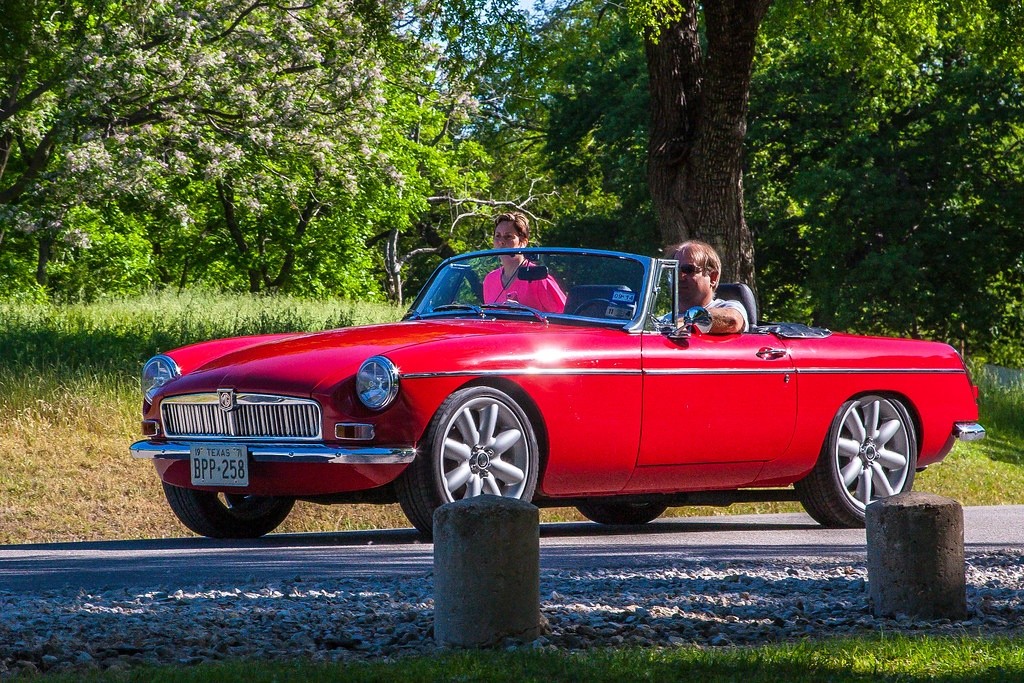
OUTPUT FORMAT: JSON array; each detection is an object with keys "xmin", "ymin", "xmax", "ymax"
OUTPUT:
[{"xmin": 673, "ymin": 265, "xmax": 705, "ymax": 276}]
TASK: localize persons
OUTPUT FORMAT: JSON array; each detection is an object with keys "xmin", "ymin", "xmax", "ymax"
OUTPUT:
[
  {"xmin": 657, "ymin": 240, "xmax": 749, "ymax": 334},
  {"xmin": 483, "ymin": 212, "xmax": 567, "ymax": 314}
]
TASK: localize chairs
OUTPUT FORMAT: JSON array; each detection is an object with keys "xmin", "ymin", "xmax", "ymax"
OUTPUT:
[
  {"xmin": 563, "ymin": 284, "xmax": 636, "ymax": 321},
  {"xmin": 715, "ymin": 283, "xmax": 757, "ymax": 333}
]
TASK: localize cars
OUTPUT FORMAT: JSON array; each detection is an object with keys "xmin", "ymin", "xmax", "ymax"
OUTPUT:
[{"xmin": 128, "ymin": 247, "xmax": 985, "ymax": 541}]
